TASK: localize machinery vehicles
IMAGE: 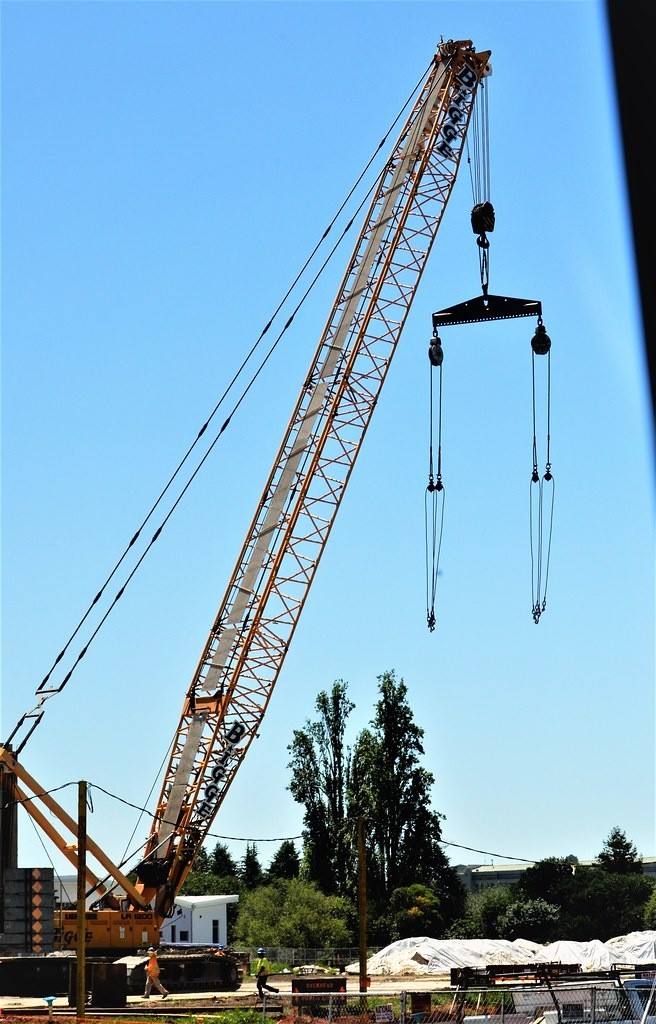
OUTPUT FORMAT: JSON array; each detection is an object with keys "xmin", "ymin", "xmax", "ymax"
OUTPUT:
[{"xmin": 1, "ymin": 31, "xmax": 549, "ymax": 997}]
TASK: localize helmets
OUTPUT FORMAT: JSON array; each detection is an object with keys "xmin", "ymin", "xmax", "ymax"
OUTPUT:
[
  {"xmin": 257, "ymin": 948, "xmax": 266, "ymax": 953},
  {"xmin": 147, "ymin": 947, "xmax": 154, "ymax": 954},
  {"xmin": 218, "ymin": 945, "xmax": 224, "ymax": 949}
]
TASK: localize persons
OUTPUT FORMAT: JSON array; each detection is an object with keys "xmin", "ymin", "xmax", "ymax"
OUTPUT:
[
  {"xmin": 141, "ymin": 947, "xmax": 169, "ymax": 998},
  {"xmin": 255, "ymin": 948, "xmax": 279, "ymax": 998},
  {"xmin": 215, "ymin": 945, "xmax": 226, "ymax": 956}
]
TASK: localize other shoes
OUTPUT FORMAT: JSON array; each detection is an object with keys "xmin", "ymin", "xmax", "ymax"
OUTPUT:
[
  {"xmin": 141, "ymin": 996, "xmax": 149, "ymax": 998},
  {"xmin": 162, "ymin": 991, "xmax": 169, "ymax": 999},
  {"xmin": 276, "ymin": 989, "xmax": 280, "ymax": 993}
]
